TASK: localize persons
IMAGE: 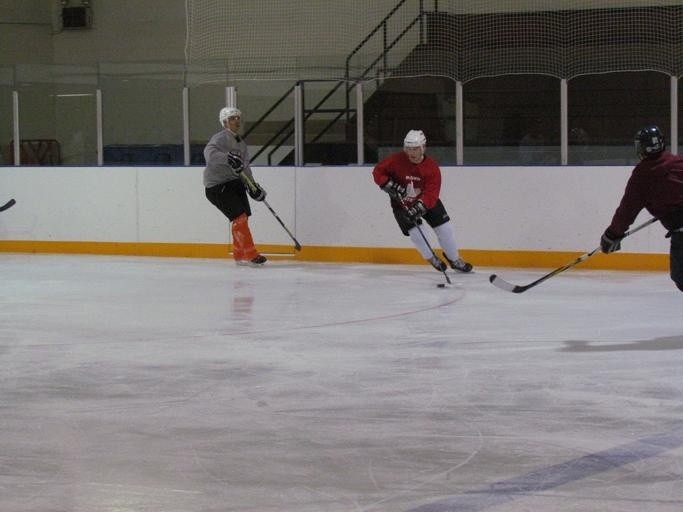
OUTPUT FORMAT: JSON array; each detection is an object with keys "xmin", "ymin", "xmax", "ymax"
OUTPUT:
[
  {"xmin": 372, "ymin": 130, "xmax": 476, "ymax": 275},
  {"xmin": 600, "ymin": 125, "xmax": 683, "ymax": 294},
  {"xmin": 202, "ymin": 106, "xmax": 270, "ymax": 268}
]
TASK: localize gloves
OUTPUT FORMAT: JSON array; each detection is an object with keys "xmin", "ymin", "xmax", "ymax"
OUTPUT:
[
  {"xmin": 383, "ymin": 179, "xmax": 407, "ymax": 200},
  {"xmin": 228, "ymin": 151, "xmax": 244, "ymax": 173},
  {"xmin": 404, "ymin": 201, "xmax": 427, "ymax": 224},
  {"xmin": 247, "ymin": 183, "xmax": 265, "ymax": 201},
  {"xmin": 601, "ymin": 230, "xmax": 621, "ymax": 254}
]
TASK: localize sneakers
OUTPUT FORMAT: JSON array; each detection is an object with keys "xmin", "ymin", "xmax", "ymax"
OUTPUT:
[
  {"xmin": 428, "ymin": 256, "xmax": 447, "ymax": 272},
  {"xmin": 443, "ymin": 251, "xmax": 472, "ymax": 272},
  {"xmin": 252, "ymin": 255, "xmax": 267, "ymax": 264}
]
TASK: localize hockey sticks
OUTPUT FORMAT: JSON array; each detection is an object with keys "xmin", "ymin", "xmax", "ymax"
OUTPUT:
[
  {"xmin": 238, "ymin": 162, "xmax": 301, "ymax": 251},
  {"xmin": 488, "ymin": 215, "xmax": 659, "ymax": 293},
  {"xmin": 404, "ymin": 196, "xmax": 452, "ymax": 288}
]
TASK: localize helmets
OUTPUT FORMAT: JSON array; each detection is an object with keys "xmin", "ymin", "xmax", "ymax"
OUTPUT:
[
  {"xmin": 634, "ymin": 126, "xmax": 666, "ymax": 160},
  {"xmin": 404, "ymin": 130, "xmax": 427, "ymax": 148},
  {"xmin": 219, "ymin": 107, "xmax": 242, "ymax": 127}
]
